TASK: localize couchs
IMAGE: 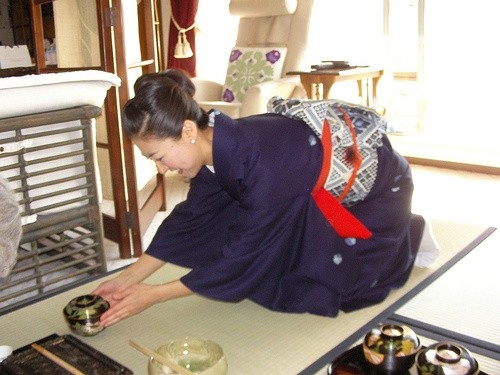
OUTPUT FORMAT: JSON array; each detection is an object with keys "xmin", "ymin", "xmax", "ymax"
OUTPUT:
[{"xmin": 190, "ymin": 0, "xmax": 314, "ymax": 119}]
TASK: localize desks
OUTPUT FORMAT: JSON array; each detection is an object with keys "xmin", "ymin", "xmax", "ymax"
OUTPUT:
[{"xmin": 285, "ymin": 68, "xmax": 385, "ymax": 106}]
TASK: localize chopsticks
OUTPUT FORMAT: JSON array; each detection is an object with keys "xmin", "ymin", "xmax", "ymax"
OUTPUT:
[
  {"xmin": 31, "ymin": 344, "xmax": 85, "ymax": 375},
  {"xmin": 128, "ymin": 339, "xmax": 195, "ymax": 375}
]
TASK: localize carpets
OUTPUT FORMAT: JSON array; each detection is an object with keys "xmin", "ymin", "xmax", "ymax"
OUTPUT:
[{"xmin": 0, "ymin": 217, "xmax": 498, "ymax": 375}]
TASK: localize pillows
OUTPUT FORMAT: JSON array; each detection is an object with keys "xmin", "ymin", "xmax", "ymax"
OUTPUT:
[{"xmin": 221, "ymin": 47, "xmax": 288, "ymax": 104}]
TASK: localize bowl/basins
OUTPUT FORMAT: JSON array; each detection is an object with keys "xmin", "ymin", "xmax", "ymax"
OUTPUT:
[
  {"xmin": 148, "ymin": 338, "xmax": 228, "ymax": 375},
  {"xmin": 415, "ymin": 341, "xmax": 480, "ymax": 375},
  {"xmin": 363, "ymin": 324, "xmax": 422, "ymax": 372},
  {"xmin": 63, "ymin": 294, "xmax": 110, "ymax": 336}
]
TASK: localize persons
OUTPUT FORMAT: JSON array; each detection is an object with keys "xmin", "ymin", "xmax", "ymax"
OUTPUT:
[
  {"xmin": 91, "ymin": 69, "xmax": 426, "ymax": 328},
  {"xmin": 0, "ymin": 172, "xmax": 22, "ymax": 364}
]
